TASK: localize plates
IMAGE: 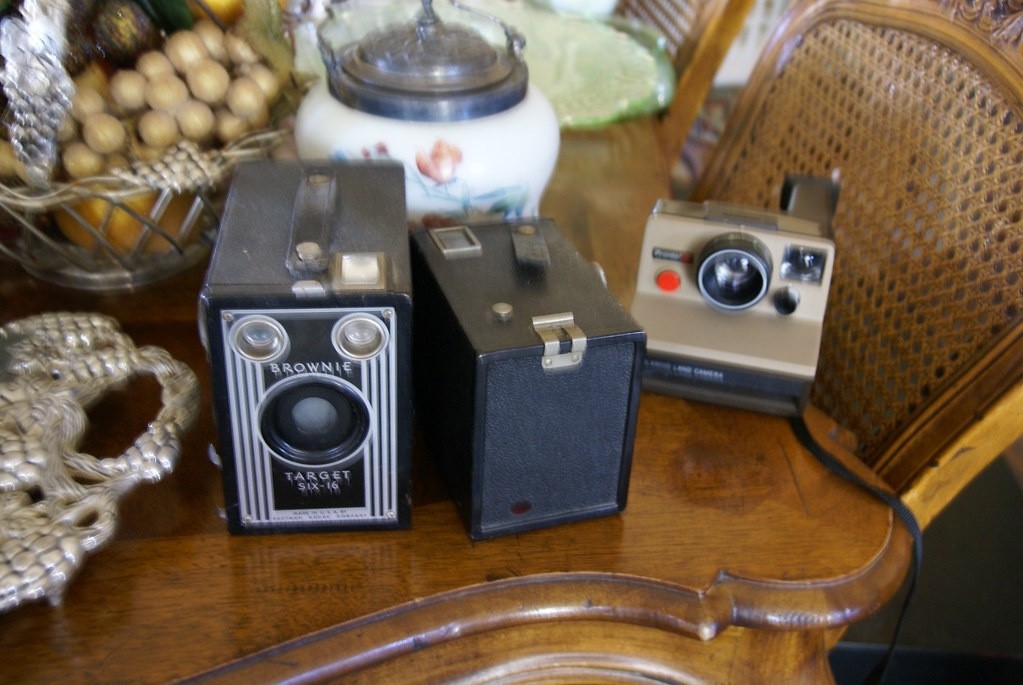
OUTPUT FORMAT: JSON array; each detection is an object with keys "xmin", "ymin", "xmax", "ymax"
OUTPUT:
[{"xmin": 563, "ymin": 18, "xmax": 678, "ymax": 127}]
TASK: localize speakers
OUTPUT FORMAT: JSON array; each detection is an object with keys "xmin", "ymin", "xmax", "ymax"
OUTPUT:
[
  {"xmin": 412, "ymin": 216, "xmax": 647, "ymax": 543},
  {"xmin": 200, "ymin": 159, "xmax": 420, "ymax": 532}
]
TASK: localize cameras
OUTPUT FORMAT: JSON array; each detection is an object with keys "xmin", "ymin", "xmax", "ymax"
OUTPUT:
[{"xmin": 630, "ymin": 171, "xmax": 840, "ymax": 422}]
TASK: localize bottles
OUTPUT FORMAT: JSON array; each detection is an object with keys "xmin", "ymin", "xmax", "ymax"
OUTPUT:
[{"xmin": 295, "ymin": 0, "xmax": 558, "ymax": 229}]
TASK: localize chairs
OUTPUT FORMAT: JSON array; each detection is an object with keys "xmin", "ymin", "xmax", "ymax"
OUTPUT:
[
  {"xmin": 687, "ymin": 0, "xmax": 1023, "ymax": 534},
  {"xmin": 608, "ymin": 0, "xmax": 756, "ymax": 163}
]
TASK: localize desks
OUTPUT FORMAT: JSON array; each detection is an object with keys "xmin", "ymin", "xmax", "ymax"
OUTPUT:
[{"xmin": 0, "ymin": 1, "xmax": 913, "ymax": 685}]
